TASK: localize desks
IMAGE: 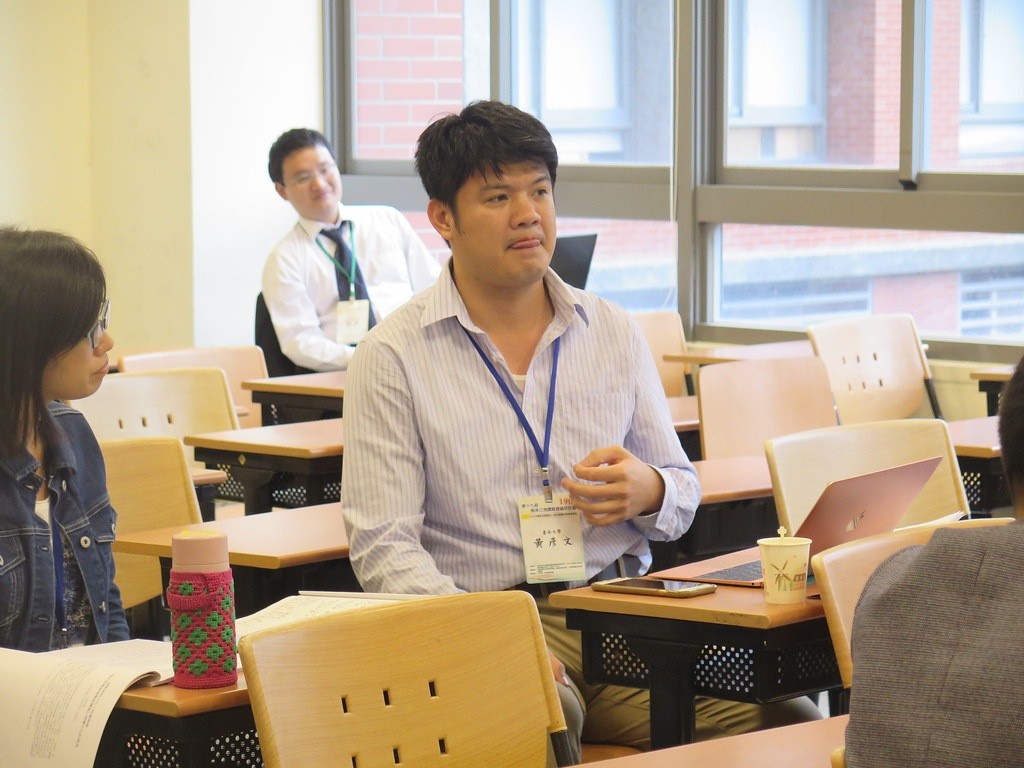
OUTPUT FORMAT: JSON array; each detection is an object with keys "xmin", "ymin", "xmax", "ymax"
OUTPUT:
[
  {"xmin": 552, "ymin": 543, "xmax": 849, "ymax": 749},
  {"xmin": 112, "ymin": 504, "xmax": 360, "ymax": 611},
  {"xmin": 945, "ymin": 413, "xmax": 1009, "ymax": 515},
  {"xmin": 665, "ymin": 395, "xmax": 700, "ymax": 462},
  {"xmin": 185, "ymin": 419, "xmax": 344, "ymax": 515},
  {"xmin": 663, "ymin": 336, "xmax": 932, "ymax": 366},
  {"xmin": 243, "ymin": 371, "xmax": 345, "ymax": 428},
  {"xmin": 680, "ymin": 457, "xmax": 780, "ymax": 563},
  {"xmin": 563, "ymin": 714, "xmax": 850, "ymax": 768},
  {"xmin": 102, "ymin": 679, "xmax": 261, "ymax": 768}
]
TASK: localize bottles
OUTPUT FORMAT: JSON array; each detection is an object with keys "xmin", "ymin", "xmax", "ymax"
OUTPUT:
[{"xmin": 167, "ymin": 531, "xmax": 238, "ymax": 689}]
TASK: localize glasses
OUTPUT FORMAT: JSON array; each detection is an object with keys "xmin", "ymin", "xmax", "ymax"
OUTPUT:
[
  {"xmin": 282, "ymin": 160, "xmax": 337, "ymax": 186},
  {"xmin": 85, "ymin": 296, "xmax": 110, "ymax": 363}
]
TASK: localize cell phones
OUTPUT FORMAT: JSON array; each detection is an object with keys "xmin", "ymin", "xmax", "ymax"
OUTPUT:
[{"xmin": 591, "ymin": 576, "xmax": 717, "ymax": 600}]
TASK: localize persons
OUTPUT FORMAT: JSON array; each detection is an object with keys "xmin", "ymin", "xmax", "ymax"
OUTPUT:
[
  {"xmin": 340, "ymin": 100, "xmax": 824, "ymax": 768},
  {"xmin": 844, "ymin": 354, "xmax": 1024, "ymax": 768},
  {"xmin": 261, "ymin": 128, "xmax": 443, "ymax": 372},
  {"xmin": 0, "ymin": 227, "xmax": 132, "ymax": 653}
]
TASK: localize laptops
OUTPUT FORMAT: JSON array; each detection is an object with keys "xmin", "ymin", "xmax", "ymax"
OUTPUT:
[
  {"xmin": 548, "ymin": 234, "xmax": 597, "ymax": 290},
  {"xmin": 693, "ymin": 455, "xmax": 945, "ymax": 588}
]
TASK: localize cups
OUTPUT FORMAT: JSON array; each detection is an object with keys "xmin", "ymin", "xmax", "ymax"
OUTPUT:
[{"xmin": 757, "ymin": 537, "xmax": 812, "ymax": 604}]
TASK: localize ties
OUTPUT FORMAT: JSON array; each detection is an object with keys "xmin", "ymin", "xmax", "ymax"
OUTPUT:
[{"xmin": 318, "ymin": 221, "xmax": 377, "ymax": 346}]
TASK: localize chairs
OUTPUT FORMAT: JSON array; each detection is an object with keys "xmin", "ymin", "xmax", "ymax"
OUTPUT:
[
  {"xmin": 811, "ymin": 517, "xmax": 1013, "ymax": 705},
  {"xmin": 119, "ymin": 345, "xmax": 270, "ymax": 429},
  {"xmin": 809, "ymin": 311, "xmax": 944, "ymax": 428},
  {"xmin": 63, "ymin": 369, "xmax": 240, "ymax": 469},
  {"xmin": 698, "ymin": 356, "xmax": 839, "ymax": 461},
  {"xmin": 632, "ymin": 310, "xmax": 691, "ymax": 400},
  {"xmin": 96, "ymin": 437, "xmax": 206, "ymax": 611},
  {"xmin": 765, "ymin": 418, "xmax": 970, "ymax": 538},
  {"xmin": 240, "ymin": 591, "xmax": 574, "ymax": 768}
]
